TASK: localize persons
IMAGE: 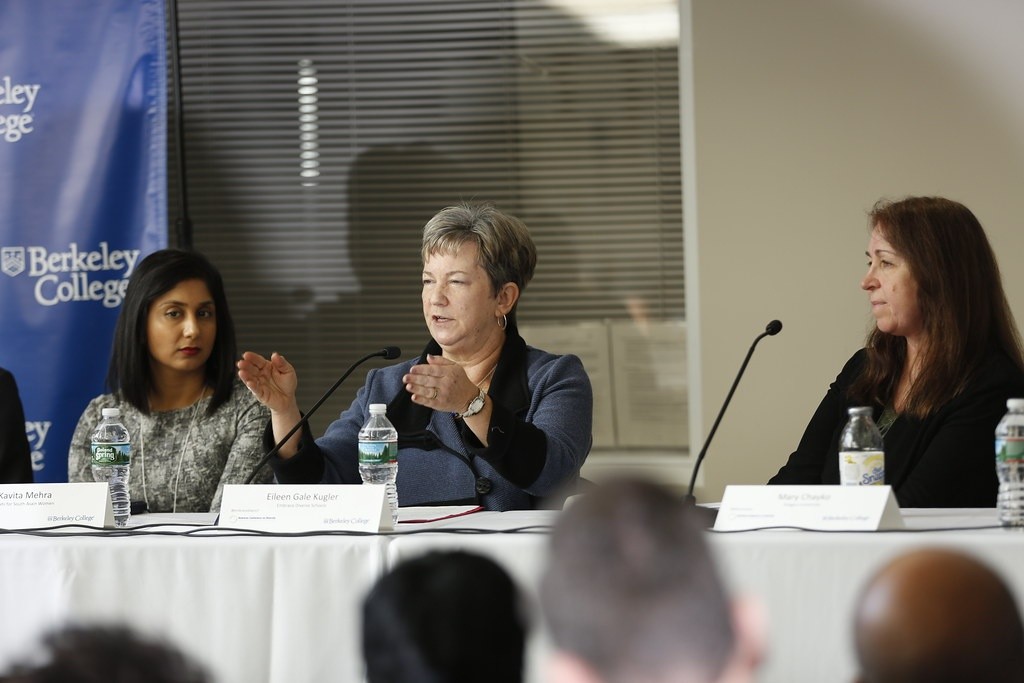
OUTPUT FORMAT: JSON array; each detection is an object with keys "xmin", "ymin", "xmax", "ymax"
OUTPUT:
[
  {"xmin": 767, "ymin": 196, "xmax": 1024, "ymax": 507},
  {"xmin": 356, "ymin": 546, "xmax": 530, "ymax": 683},
  {"xmin": 237, "ymin": 205, "xmax": 595, "ymax": 510},
  {"xmin": 851, "ymin": 549, "xmax": 1024, "ymax": 683},
  {"xmin": 0, "ymin": 618, "xmax": 217, "ymax": 683},
  {"xmin": 535, "ymin": 469, "xmax": 771, "ymax": 683},
  {"xmin": 0, "ymin": 366, "xmax": 36, "ymax": 484},
  {"xmin": 68, "ymin": 248, "xmax": 277, "ymax": 514}
]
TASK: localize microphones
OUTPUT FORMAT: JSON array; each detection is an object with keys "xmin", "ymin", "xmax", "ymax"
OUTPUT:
[
  {"xmin": 243, "ymin": 346, "xmax": 402, "ymax": 485},
  {"xmin": 684, "ymin": 319, "xmax": 783, "ymax": 527}
]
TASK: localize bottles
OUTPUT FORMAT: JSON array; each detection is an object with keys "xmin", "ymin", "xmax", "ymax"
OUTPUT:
[
  {"xmin": 994, "ymin": 398, "xmax": 1024, "ymax": 527},
  {"xmin": 839, "ymin": 406, "xmax": 883, "ymax": 486},
  {"xmin": 358, "ymin": 404, "xmax": 398, "ymax": 527},
  {"xmin": 91, "ymin": 408, "xmax": 131, "ymax": 528}
]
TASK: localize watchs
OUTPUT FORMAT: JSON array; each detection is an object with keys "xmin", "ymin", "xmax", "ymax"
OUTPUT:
[{"xmin": 454, "ymin": 387, "xmax": 485, "ymax": 422}]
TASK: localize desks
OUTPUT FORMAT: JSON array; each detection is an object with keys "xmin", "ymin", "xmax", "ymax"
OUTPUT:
[{"xmin": 1, "ymin": 512, "xmax": 1024, "ymax": 683}]
